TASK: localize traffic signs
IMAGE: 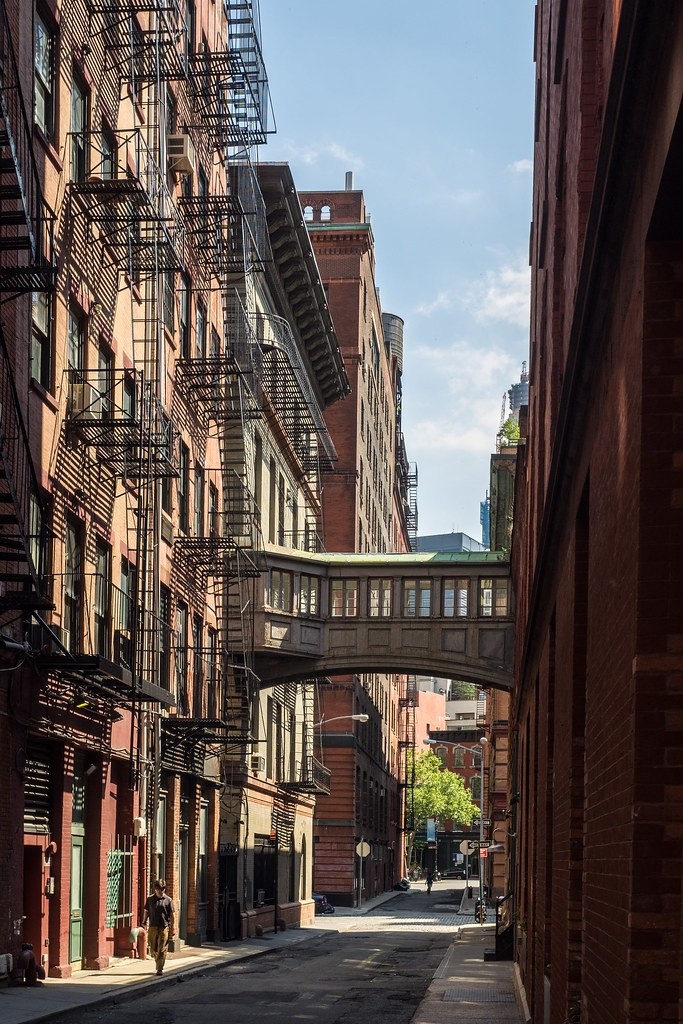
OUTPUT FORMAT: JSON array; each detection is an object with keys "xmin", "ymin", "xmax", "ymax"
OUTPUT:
[
  {"xmin": 468, "ymin": 840, "xmax": 491, "ymax": 848},
  {"xmin": 472, "ymin": 819, "xmax": 492, "ymax": 827}
]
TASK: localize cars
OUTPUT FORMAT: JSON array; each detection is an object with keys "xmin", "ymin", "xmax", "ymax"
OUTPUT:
[
  {"xmin": 438, "ymin": 866, "xmax": 470, "ymax": 880},
  {"xmin": 312, "ymin": 894, "xmax": 328, "ymax": 914}
]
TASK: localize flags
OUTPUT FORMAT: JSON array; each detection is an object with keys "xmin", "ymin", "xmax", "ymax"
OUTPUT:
[{"xmin": 426, "ymin": 818, "xmax": 436, "ymax": 842}]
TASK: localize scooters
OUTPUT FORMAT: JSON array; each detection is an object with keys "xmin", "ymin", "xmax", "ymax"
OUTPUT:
[{"xmin": 473, "ymin": 897, "xmax": 487, "ymax": 924}]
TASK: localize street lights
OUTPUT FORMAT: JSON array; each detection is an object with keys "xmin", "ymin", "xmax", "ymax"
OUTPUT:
[{"xmin": 422, "ymin": 736, "xmax": 483, "ymax": 925}]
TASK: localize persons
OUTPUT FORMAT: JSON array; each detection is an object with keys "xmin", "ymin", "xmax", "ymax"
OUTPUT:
[
  {"xmin": 425, "ymin": 873, "xmax": 433, "ymax": 895},
  {"xmin": 142, "ymin": 879, "xmax": 175, "ymax": 976}
]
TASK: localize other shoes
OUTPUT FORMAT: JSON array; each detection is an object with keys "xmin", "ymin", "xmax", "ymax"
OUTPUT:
[{"xmin": 157, "ymin": 970, "xmax": 163, "ymax": 975}]
